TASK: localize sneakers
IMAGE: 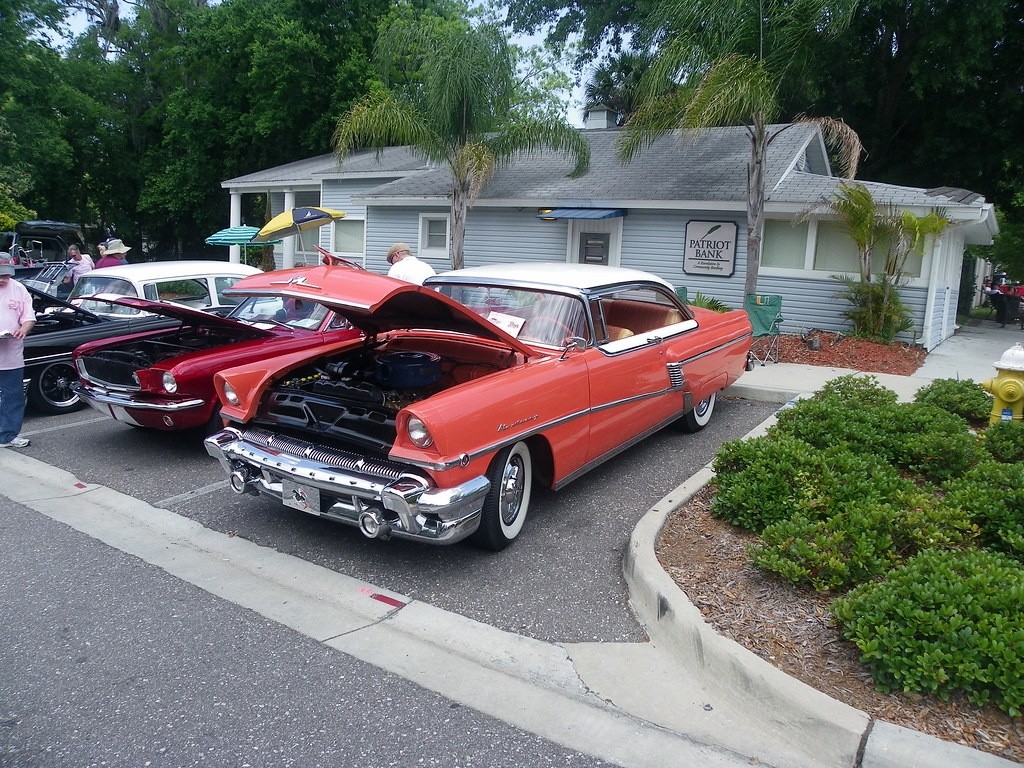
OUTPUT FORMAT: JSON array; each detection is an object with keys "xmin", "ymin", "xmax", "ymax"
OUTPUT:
[{"xmin": 0, "ymin": 436, "xmax": 31, "ymax": 448}]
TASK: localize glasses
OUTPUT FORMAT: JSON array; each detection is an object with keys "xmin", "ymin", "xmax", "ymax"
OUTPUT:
[
  {"xmin": 988, "ymin": 283, "xmax": 992, "ymax": 284},
  {"xmin": 391, "ymin": 256, "xmax": 393, "ymax": 265},
  {"xmin": 69, "ymin": 253, "xmax": 77, "ymax": 258}
]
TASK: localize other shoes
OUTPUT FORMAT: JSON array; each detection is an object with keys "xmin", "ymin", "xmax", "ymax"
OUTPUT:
[{"xmin": 1014, "ymin": 318, "xmax": 1020, "ymax": 320}]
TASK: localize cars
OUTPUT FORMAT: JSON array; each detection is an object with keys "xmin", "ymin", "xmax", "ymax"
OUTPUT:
[
  {"xmin": 1, "ymin": 219, "xmax": 88, "ymax": 303},
  {"xmin": 203, "ymin": 260, "xmax": 753, "ymax": 552},
  {"xmin": 71, "ymin": 295, "xmax": 367, "ymax": 432},
  {"xmin": 23, "ymin": 260, "xmax": 266, "ymax": 416}
]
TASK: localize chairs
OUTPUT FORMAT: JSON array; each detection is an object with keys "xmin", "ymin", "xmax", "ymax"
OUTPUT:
[
  {"xmin": 588, "ymin": 323, "xmax": 635, "ymax": 343},
  {"xmin": 741, "ymin": 294, "xmax": 785, "ymax": 366}
]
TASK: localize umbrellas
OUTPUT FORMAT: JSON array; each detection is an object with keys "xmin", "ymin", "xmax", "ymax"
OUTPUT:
[
  {"xmin": 205, "ymin": 224, "xmax": 283, "ymax": 266},
  {"xmin": 250, "ymin": 206, "xmax": 348, "ymax": 268}
]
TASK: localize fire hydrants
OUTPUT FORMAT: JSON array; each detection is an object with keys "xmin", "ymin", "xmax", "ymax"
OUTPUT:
[{"xmin": 981, "ymin": 339, "xmax": 1024, "ymax": 426}]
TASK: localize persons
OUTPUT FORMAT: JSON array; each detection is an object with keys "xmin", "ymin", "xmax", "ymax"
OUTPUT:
[
  {"xmin": 386, "ymin": 243, "xmax": 437, "ymax": 287},
  {"xmin": 60, "ymin": 237, "xmax": 132, "ymax": 286},
  {"xmin": 0, "ymin": 251, "xmax": 38, "ymax": 448}
]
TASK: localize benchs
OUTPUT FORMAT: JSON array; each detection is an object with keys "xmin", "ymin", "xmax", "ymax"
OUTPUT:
[
  {"xmin": 201, "ymin": 294, "xmax": 241, "ymax": 305},
  {"xmin": 588, "ymin": 298, "xmax": 679, "ymax": 336}
]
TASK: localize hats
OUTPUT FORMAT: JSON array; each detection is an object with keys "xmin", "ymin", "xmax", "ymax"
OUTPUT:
[
  {"xmin": 387, "ymin": 243, "xmax": 410, "ymax": 263},
  {"xmin": 0, "ymin": 252, "xmax": 15, "ymax": 276},
  {"xmin": 103, "ymin": 239, "xmax": 132, "ymax": 254},
  {"xmin": 100, "ymin": 242, "xmax": 108, "ymax": 249},
  {"xmin": 106, "ymin": 238, "xmax": 115, "ymax": 243}
]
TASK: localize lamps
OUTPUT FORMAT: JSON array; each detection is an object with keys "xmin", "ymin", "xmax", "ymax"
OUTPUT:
[{"xmin": 537, "ymin": 208, "xmax": 557, "ymax": 220}]
